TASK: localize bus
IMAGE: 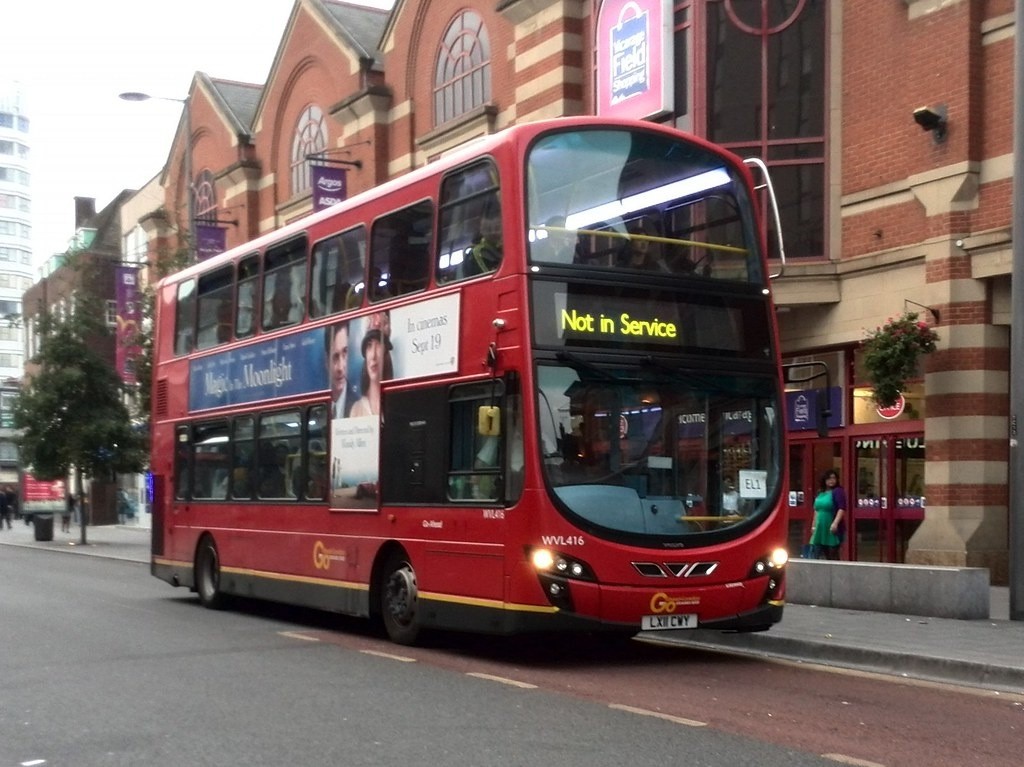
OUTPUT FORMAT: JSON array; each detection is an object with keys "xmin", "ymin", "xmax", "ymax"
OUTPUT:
[{"xmin": 145, "ymin": 117, "xmax": 836, "ymax": 649}]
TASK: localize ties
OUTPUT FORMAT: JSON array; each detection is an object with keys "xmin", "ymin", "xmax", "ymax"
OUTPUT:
[{"xmin": 332, "ymin": 405, "xmax": 337, "ymax": 419}]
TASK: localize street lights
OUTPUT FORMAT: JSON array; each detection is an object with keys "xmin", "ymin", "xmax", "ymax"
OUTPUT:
[{"xmin": 119, "ymin": 88, "xmax": 197, "ymax": 264}]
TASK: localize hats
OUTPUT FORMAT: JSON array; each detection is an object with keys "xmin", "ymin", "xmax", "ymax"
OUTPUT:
[{"xmin": 360, "ymin": 309, "xmax": 393, "ymax": 356}]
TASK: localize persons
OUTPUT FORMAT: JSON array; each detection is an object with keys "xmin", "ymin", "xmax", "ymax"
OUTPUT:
[
  {"xmin": 348, "ymin": 310, "xmax": 395, "ymax": 422},
  {"xmin": 531, "ymin": 216, "xmax": 587, "ymax": 263},
  {"xmin": 0, "ymin": 484, "xmax": 24, "ymax": 532},
  {"xmin": 616, "ymin": 229, "xmax": 660, "ymax": 272},
  {"xmin": 60, "ymin": 492, "xmax": 77, "ymax": 530},
  {"xmin": 323, "ymin": 320, "xmax": 370, "ymax": 420},
  {"xmin": 181, "ymin": 442, "xmax": 327, "ymax": 500},
  {"xmin": 462, "ymin": 208, "xmax": 507, "ymax": 278},
  {"xmin": 806, "ymin": 468, "xmax": 848, "ymax": 561},
  {"xmin": 721, "ymin": 475, "xmax": 745, "ymax": 514},
  {"xmin": 116, "ymin": 488, "xmax": 129, "ymax": 524}
]
